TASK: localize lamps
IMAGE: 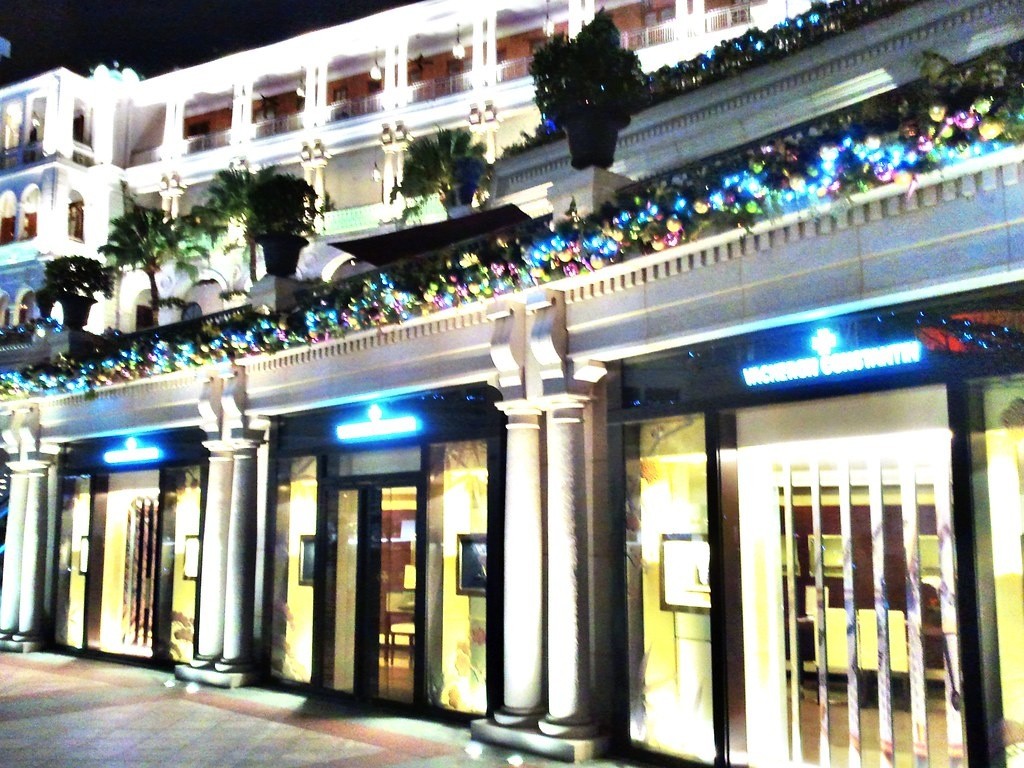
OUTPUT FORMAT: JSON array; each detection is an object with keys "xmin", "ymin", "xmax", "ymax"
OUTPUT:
[
  {"xmin": 157, "ymin": 29, "xmax": 496, "ymax": 189},
  {"xmin": 543, "ymin": 13, "xmax": 555, "ymax": 37}
]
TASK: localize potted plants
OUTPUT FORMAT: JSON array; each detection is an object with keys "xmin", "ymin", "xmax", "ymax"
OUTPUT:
[
  {"xmin": 243, "ymin": 176, "xmax": 319, "ymax": 276},
  {"xmin": 528, "ymin": 9, "xmax": 653, "ymax": 168},
  {"xmin": 401, "ymin": 127, "xmax": 490, "ymax": 220},
  {"xmin": 35, "ymin": 256, "xmax": 117, "ymax": 329}
]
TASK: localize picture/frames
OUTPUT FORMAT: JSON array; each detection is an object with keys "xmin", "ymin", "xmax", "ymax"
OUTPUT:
[
  {"xmin": 78, "ymin": 534, "xmax": 88, "ymax": 575},
  {"xmin": 782, "ymin": 534, "xmax": 845, "ymax": 580},
  {"xmin": 455, "ymin": 532, "xmax": 486, "ymax": 598},
  {"xmin": 299, "ymin": 534, "xmax": 315, "ymax": 586},
  {"xmin": 659, "ymin": 531, "xmax": 711, "ymax": 614},
  {"xmin": 183, "ymin": 534, "xmax": 199, "ymax": 581}
]
